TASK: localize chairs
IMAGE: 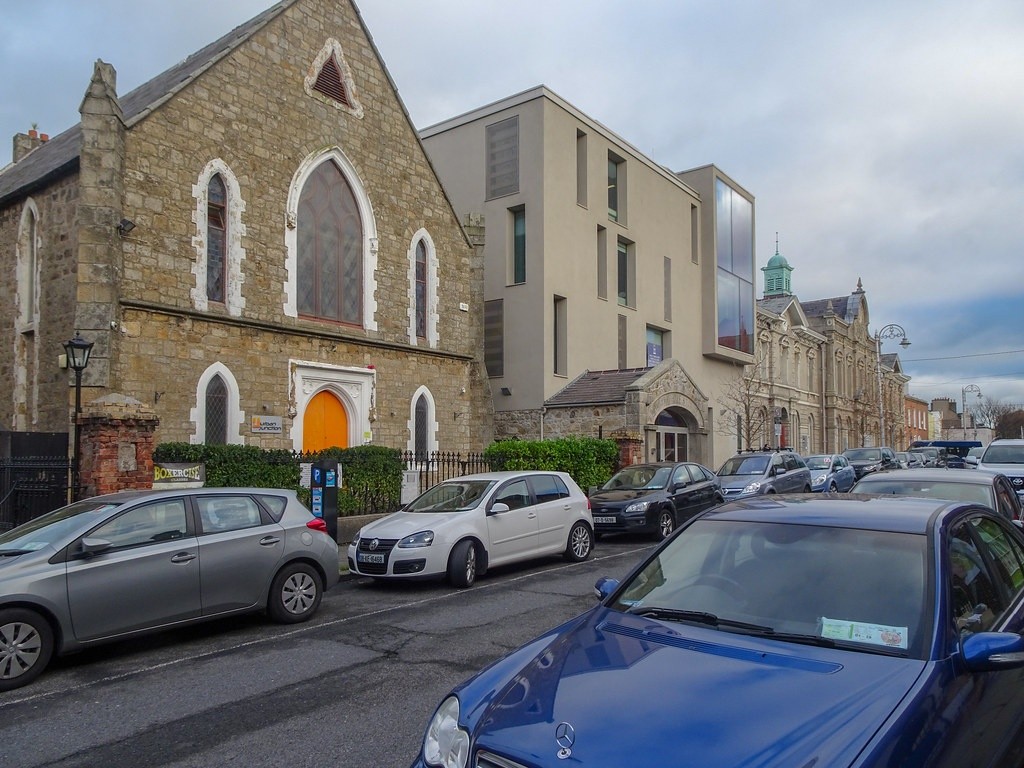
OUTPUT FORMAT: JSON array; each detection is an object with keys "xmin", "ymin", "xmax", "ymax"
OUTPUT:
[{"xmin": 719, "ymin": 540, "xmax": 922, "ymax": 628}]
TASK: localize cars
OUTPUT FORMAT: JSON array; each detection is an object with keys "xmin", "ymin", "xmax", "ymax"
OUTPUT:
[
  {"xmin": 841, "ymin": 447, "xmax": 903, "ymax": 481},
  {"xmin": 801, "ymin": 455, "xmax": 858, "ymax": 493},
  {"xmin": 0, "ymin": 487, "xmax": 339, "ymax": 693},
  {"xmin": 715, "ymin": 445, "xmax": 812, "ymax": 502},
  {"xmin": 409, "ymin": 488, "xmax": 1024, "ymax": 767},
  {"xmin": 846, "ymin": 466, "xmax": 1023, "ymax": 533},
  {"xmin": 894, "ymin": 439, "xmax": 986, "ymax": 470},
  {"xmin": 347, "ymin": 471, "xmax": 594, "ymax": 589},
  {"xmin": 588, "ymin": 461, "xmax": 724, "ymax": 543}
]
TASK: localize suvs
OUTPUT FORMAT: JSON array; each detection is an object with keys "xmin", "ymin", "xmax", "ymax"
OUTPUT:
[{"xmin": 965, "ymin": 436, "xmax": 1024, "ymax": 507}]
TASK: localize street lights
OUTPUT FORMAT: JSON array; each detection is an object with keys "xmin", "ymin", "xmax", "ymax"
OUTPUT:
[
  {"xmin": 961, "ymin": 383, "xmax": 983, "ymax": 440},
  {"xmin": 873, "ymin": 324, "xmax": 912, "ymax": 448},
  {"xmin": 60, "ymin": 329, "xmax": 95, "ymax": 503}
]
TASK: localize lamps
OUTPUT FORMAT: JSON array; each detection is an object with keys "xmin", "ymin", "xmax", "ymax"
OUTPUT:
[{"xmin": 116, "ymin": 219, "xmax": 135, "ymax": 238}]
